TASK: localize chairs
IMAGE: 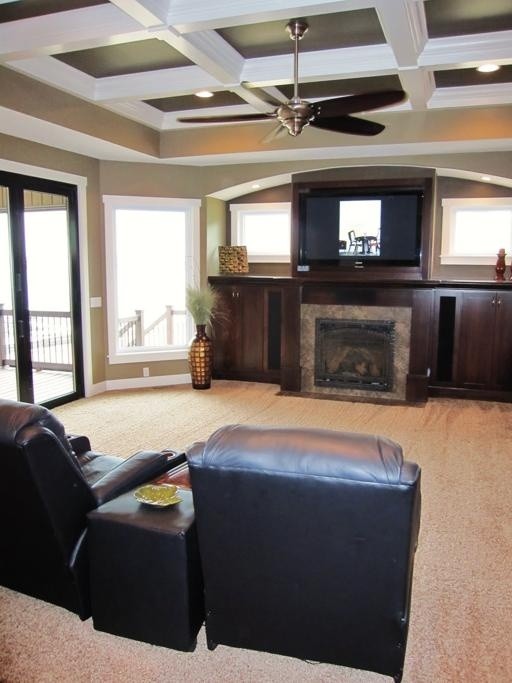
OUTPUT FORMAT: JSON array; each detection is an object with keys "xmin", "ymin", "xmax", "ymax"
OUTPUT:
[
  {"xmin": 186, "ymin": 424, "xmax": 422, "ymax": 683},
  {"xmin": 0, "ymin": 399, "xmax": 185, "ymax": 620}
]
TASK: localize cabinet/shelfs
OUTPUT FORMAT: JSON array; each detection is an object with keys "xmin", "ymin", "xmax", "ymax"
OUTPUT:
[
  {"xmin": 211, "ymin": 273, "xmax": 268, "ymax": 384},
  {"xmin": 451, "ymin": 284, "xmax": 512, "ymax": 403}
]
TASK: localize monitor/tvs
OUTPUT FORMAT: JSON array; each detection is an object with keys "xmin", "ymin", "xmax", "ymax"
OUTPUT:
[{"xmin": 298, "ymin": 187, "xmax": 425, "ymax": 273}]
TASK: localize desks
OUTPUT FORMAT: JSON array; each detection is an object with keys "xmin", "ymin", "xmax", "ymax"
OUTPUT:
[{"xmin": 86, "ymin": 462, "xmax": 200, "ymax": 656}]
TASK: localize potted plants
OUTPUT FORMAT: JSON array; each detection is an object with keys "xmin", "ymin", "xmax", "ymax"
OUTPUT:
[{"xmin": 185, "ymin": 275, "xmax": 233, "ymax": 390}]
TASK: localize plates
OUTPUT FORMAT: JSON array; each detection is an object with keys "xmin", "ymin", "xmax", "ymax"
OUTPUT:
[{"xmin": 134, "ymin": 485, "xmax": 183, "ymax": 509}]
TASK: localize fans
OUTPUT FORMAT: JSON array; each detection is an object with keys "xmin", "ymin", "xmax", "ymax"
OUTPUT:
[{"xmin": 176, "ymin": 20, "xmax": 408, "ymax": 144}]
